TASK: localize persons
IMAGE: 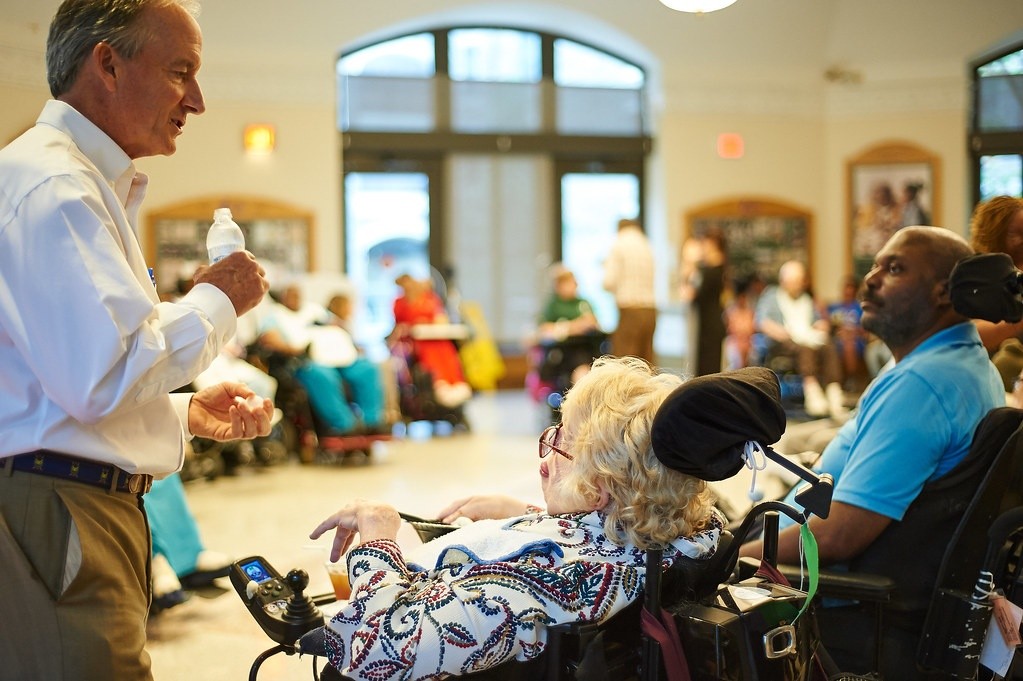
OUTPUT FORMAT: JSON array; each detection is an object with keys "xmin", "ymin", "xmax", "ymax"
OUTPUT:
[
  {"xmin": 1, "ymin": 0, "xmax": 272, "ymax": 678},
  {"xmin": 964, "ymin": 195, "xmax": 1023, "ymax": 392},
  {"xmin": 525, "ymin": 263, "xmax": 608, "ymax": 399},
  {"xmin": 601, "ymin": 220, "xmax": 659, "ymax": 366},
  {"xmin": 738, "ymin": 226, "xmax": 1008, "ymax": 570},
  {"xmin": 308, "ymin": 356, "xmax": 734, "ymax": 681},
  {"xmin": 850, "ymin": 171, "xmax": 928, "ymax": 260},
  {"xmin": 163, "ymin": 263, "xmax": 500, "ymax": 472},
  {"xmin": 677, "ymin": 230, "xmax": 868, "ymax": 428}
]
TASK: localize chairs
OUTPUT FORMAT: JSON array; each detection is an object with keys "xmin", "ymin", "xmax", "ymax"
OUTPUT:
[
  {"xmin": 717, "ymin": 252, "xmax": 1021, "ymax": 679},
  {"xmin": 166, "ymin": 289, "xmax": 860, "ymax": 482},
  {"xmin": 224, "ymin": 367, "xmax": 859, "ymax": 681}
]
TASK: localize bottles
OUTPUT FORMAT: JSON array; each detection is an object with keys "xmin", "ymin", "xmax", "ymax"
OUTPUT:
[{"xmin": 205, "ymin": 207, "xmax": 245, "ymax": 267}]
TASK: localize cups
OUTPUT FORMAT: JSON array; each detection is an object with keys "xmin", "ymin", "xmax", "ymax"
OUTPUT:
[{"xmin": 326, "ymin": 562, "xmax": 352, "ymax": 601}]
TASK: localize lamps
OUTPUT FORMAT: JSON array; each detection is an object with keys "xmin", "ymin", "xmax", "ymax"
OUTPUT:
[{"xmin": 659, "ymin": 0, "xmax": 736, "ymax": 17}]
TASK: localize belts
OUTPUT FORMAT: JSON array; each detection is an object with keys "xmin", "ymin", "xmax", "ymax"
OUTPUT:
[{"xmin": 0, "ymin": 448, "xmax": 155, "ymax": 496}]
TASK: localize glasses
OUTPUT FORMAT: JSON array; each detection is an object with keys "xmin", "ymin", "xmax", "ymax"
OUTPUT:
[{"xmin": 539, "ymin": 421, "xmax": 574, "ymax": 461}]
